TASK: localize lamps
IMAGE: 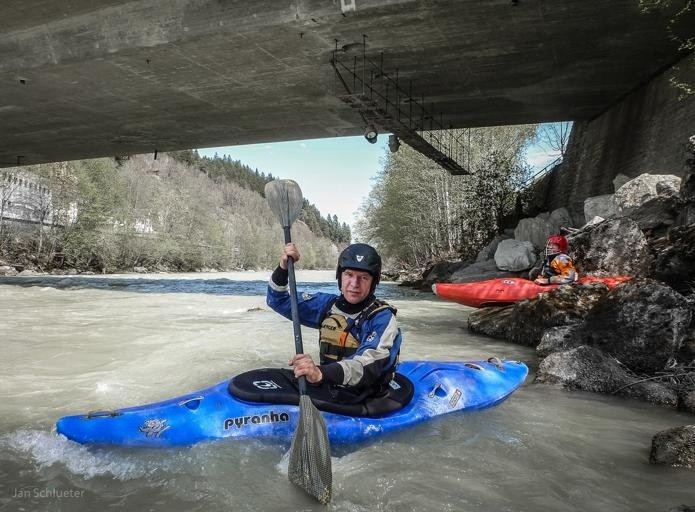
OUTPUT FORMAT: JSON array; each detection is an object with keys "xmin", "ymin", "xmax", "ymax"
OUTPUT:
[
  {"xmin": 388, "ymin": 135, "xmax": 400, "ymax": 152},
  {"xmin": 364, "ymin": 124, "xmax": 378, "ymax": 145}
]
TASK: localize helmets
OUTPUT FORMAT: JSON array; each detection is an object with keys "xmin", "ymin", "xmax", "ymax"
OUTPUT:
[
  {"xmin": 545, "ymin": 236, "xmax": 568, "ymax": 256},
  {"xmin": 336, "ymin": 244, "xmax": 382, "ymax": 285}
]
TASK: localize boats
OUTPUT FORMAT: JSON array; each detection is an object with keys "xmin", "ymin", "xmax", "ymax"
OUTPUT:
[
  {"xmin": 54, "ymin": 357, "xmax": 530, "ymax": 452},
  {"xmin": 432, "ymin": 276, "xmax": 636, "ymax": 308}
]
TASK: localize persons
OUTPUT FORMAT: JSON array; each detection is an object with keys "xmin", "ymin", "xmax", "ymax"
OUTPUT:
[
  {"xmin": 266, "ymin": 242, "xmax": 401, "ymax": 405},
  {"xmin": 534, "ymin": 234, "xmax": 579, "ymax": 286}
]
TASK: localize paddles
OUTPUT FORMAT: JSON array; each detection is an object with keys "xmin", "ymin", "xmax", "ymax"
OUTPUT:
[{"xmin": 264, "ymin": 179, "xmax": 331, "ymax": 505}]
